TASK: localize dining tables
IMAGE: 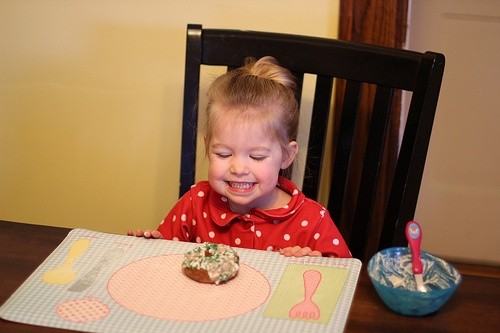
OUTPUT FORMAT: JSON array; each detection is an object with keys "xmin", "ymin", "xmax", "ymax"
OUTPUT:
[{"xmin": 0, "ymin": 219, "xmax": 500, "ymax": 333}]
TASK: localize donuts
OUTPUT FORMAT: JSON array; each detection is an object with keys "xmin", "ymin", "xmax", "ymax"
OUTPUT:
[{"xmin": 181, "ymin": 242, "xmax": 241, "ymax": 285}]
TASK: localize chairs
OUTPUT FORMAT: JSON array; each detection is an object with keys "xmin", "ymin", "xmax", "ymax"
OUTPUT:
[{"xmin": 178, "ymin": 24, "xmax": 445, "ymax": 263}]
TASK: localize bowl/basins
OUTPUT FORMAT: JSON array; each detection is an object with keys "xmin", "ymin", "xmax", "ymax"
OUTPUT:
[{"xmin": 368, "ymin": 247, "xmax": 462, "ymax": 316}]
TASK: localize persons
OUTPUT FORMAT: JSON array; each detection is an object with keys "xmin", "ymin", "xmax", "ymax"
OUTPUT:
[{"xmin": 126, "ymin": 56, "xmax": 354, "ymax": 258}]
